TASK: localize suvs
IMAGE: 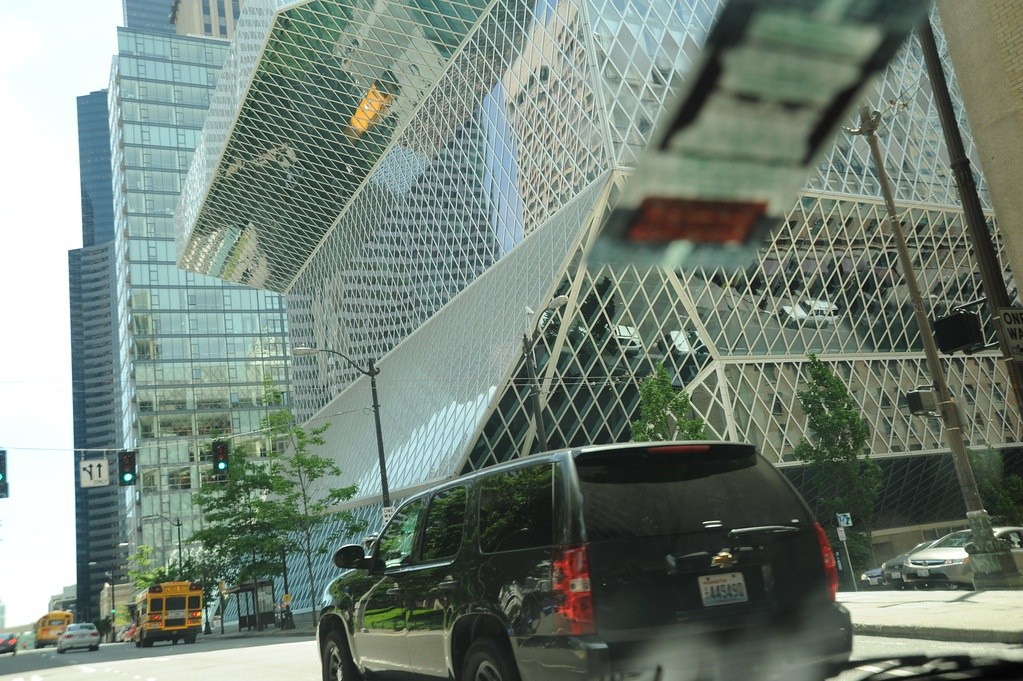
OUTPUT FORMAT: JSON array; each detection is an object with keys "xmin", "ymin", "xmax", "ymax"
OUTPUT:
[
  {"xmin": 605, "ymin": 324, "xmax": 642, "ymax": 359},
  {"xmin": 662, "ymin": 329, "xmax": 709, "ymax": 361},
  {"xmin": 316, "ymin": 439, "xmax": 853, "ymax": 681},
  {"xmin": 778, "ymin": 299, "xmax": 838, "ymax": 329}
]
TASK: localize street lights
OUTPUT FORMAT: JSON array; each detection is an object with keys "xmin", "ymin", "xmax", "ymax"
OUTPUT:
[
  {"xmin": 120, "ymin": 543, "xmax": 140, "ymax": 584},
  {"xmin": 89, "ymin": 561, "xmax": 115, "ymax": 642},
  {"xmin": 520, "ymin": 294, "xmax": 569, "ymax": 454},
  {"xmin": 291, "ymin": 346, "xmax": 391, "ymax": 508},
  {"xmin": 137, "ymin": 513, "xmax": 183, "ymax": 580}
]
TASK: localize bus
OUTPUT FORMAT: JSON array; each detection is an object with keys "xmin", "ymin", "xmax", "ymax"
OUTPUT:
[
  {"xmin": 129, "ymin": 581, "xmax": 203, "ymax": 647},
  {"xmin": 34, "ymin": 612, "xmax": 73, "ymax": 650}
]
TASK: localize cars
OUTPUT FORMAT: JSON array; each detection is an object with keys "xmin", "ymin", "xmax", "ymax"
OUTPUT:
[
  {"xmin": 0, "ymin": 633, "xmax": 18, "ymax": 656},
  {"xmin": 861, "ymin": 538, "xmax": 966, "ymax": 593},
  {"xmin": 57, "ymin": 622, "xmax": 101, "ymax": 653},
  {"xmin": 901, "ymin": 525, "xmax": 1023, "ymax": 591},
  {"xmin": 18, "ymin": 631, "xmax": 36, "ymax": 651}
]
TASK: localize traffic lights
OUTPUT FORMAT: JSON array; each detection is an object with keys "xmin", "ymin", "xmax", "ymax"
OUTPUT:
[
  {"xmin": 931, "ymin": 311, "xmax": 985, "ymax": 357},
  {"xmin": 211, "ymin": 440, "xmax": 230, "ymax": 476},
  {"xmin": 117, "ymin": 450, "xmax": 136, "ymax": 486},
  {"xmin": 0, "ymin": 450, "xmax": 9, "ymax": 495}
]
